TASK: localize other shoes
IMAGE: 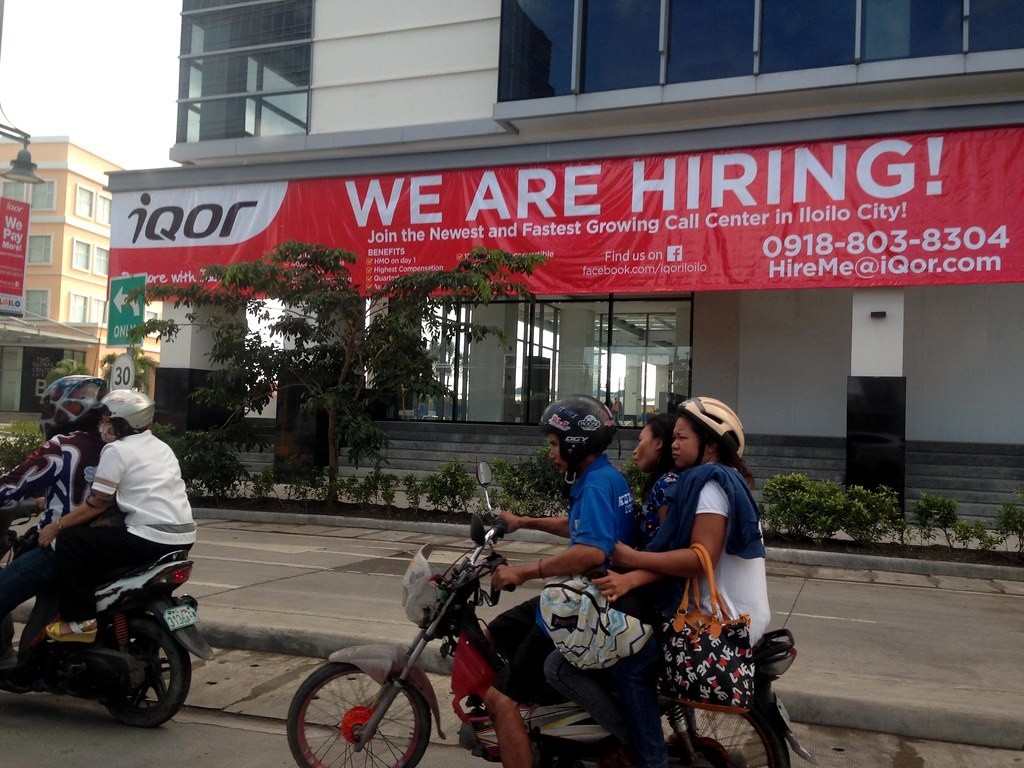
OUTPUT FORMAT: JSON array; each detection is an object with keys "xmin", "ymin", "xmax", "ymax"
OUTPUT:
[{"xmin": 0, "ymin": 646, "xmax": 17, "ymax": 669}]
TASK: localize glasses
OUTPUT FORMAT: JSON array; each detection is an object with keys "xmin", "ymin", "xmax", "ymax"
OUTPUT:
[{"xmin": 98, "ymin": 419, "xmax": 110, "ymax": 426}]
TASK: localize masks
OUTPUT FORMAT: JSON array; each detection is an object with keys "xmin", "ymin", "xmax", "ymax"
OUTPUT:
[{"xmin": 101, "ymin": 425, "xmax": 116, "ymax": 443}]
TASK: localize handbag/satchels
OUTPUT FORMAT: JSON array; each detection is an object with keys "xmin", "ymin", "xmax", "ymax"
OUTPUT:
[
  {"xmin": 540, "ymin": 564, "xmax": 654, "ymax": 670},
  {"xmin": 655, "ymin": 543, "xmax": 755, "ymax": 713}
]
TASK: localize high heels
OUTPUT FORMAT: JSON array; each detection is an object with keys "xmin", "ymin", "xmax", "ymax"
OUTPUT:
[{"xmin": 45, "ymin": 619, "xmax": 98, "ymax": 642}]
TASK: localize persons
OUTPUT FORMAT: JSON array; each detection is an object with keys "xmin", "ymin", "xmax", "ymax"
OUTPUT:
[
  {"xmin": 0, "ymin": 374, "xmax": 196, "ymax": 674},
  {"xmin": 482, "ymin": 395, "xmax": 769, "ymax": 768}
]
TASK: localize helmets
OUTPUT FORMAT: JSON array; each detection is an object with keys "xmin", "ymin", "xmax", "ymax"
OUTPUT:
[
  {"xmin": 678, "ymin": 398, "xmax": 744, "ymax": 459},
  {"xmin": 100, "ymin": 389, "xmax": 155, "ymax": 429},
  {"xmin": 539, "ymin": 393, "xmax": 618, "ymax": 464},
  {"xmin": 39, "ymin": 375, "xmax": 108, "ymax": 441}
]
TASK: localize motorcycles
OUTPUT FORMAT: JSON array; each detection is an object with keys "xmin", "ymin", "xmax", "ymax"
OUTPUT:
[
  {"xmin": 1, "ymin": 484, "xmax": 215, "ymax": 730},
  {"xmin": 284, "ymin": 460, "xmax": 819, "ymax": 767}
]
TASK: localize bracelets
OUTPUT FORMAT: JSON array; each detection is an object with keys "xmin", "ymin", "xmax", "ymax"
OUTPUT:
[
  {"xmin": 57, "ymin": 519, "xmax": 62, "ymax": 531},
  {"xmin": 538, "ymin": 559, "xmax": 545, "ymax": 579}
]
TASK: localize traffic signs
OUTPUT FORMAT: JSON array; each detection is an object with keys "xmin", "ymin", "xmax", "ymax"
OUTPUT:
[{"xmin": 106, "ymin": 272, "xmax": 146, "ymax": 348}]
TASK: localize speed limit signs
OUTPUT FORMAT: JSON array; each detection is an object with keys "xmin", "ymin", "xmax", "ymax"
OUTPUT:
[{"xmin": 110, "ymin": 353, "xmax": 135, "ymax": 392}]
